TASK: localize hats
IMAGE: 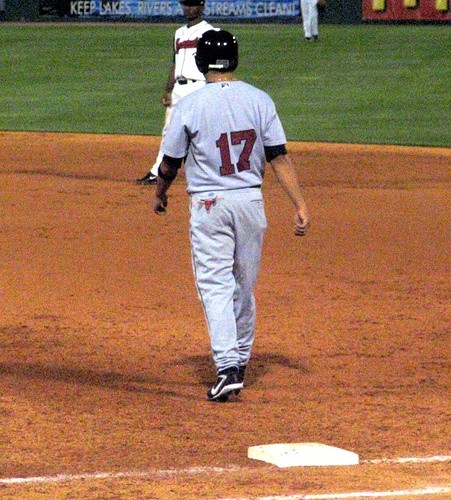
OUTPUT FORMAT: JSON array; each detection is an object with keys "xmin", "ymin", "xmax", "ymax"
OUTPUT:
[{"xmin": 193, "ymin": 28, "xmax": 239, "ymax": 73}]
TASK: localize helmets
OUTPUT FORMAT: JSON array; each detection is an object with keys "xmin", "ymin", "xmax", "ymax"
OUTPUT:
[{"xmin": 179, "ymin": 0, "xmax": 205, "ymax": 6}]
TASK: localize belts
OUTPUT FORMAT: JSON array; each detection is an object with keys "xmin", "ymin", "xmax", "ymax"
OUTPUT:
[{"xmin": 178, "ymin": 80, "xmax": 196, "ymax": 84}]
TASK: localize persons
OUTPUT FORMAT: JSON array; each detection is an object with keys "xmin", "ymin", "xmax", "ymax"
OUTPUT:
[
  {"xmin": 136, "ymin": 0, "xmax": 217, "ymax": 184},
  {"xmin": 300, "ymin": 0, "xmax": 319, "ymax": 41},
  {"xmin": 153, "ymin": 28, "xmax": 311, "ymax": 402}
]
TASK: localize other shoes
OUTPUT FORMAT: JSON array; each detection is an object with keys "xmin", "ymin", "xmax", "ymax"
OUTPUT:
[
  {"xmin": 137, "ymin": 172, "xmax": 157, "ymax": 185},
  {"xmin": 207, "ymin": 369, "xmax": 245, "ymax": 401},
  {"xmin": 305, "ymin": 37, "xmax": 312, "ymax": 42},
  {"xmin": 313, "ymin": 35, "xmax": 318, "ymax": 39},
  {"xmin": 216, "ymin": 371, "xmax": 245, "ymax": 402}
]
